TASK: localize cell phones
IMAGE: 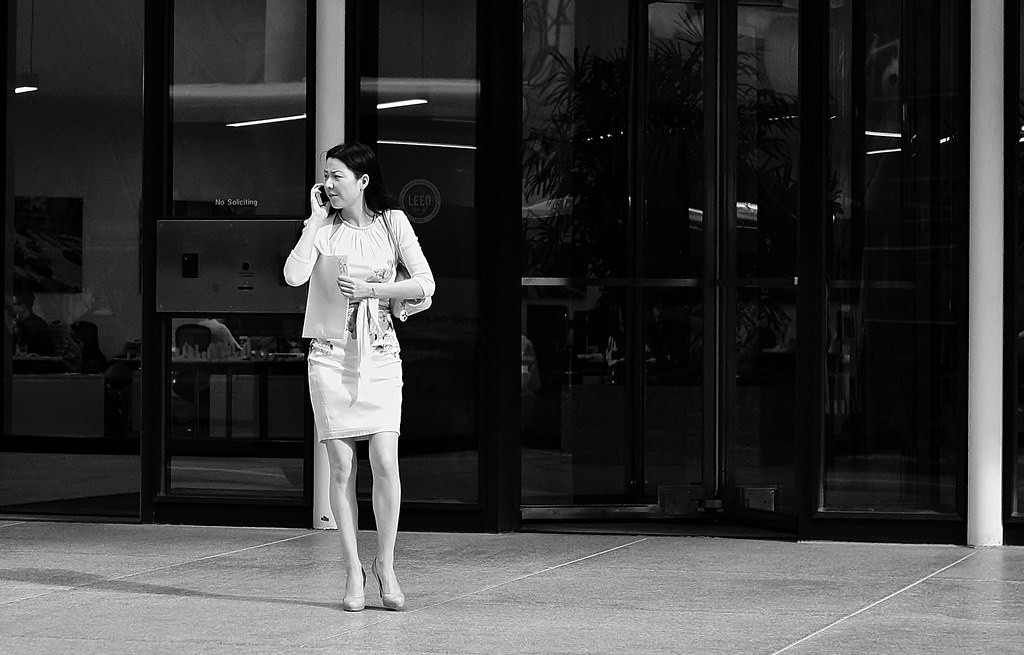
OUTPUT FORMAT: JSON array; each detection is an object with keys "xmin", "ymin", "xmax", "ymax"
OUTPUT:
[{"xmin": 316, "ymin": 186, "xmax": 330, "ymax": 207}]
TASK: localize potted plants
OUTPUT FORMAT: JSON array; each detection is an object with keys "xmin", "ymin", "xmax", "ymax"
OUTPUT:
[{"xmin": 521, "ymin": 11, "xmax": 844, "ymax": 494}]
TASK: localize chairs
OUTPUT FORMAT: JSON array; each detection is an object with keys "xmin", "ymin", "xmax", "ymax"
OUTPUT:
[
  {"xmin": 64, "ymin": 321, "xmax": 129, "ymax": 436},
  {"xmin": 48, "ymin": 320, "xmax": 72, "ymax": 357}
]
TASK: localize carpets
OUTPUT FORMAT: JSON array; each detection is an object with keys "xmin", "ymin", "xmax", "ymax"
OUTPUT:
[{"xmin": 0, "ymin": 488, "xmax": 371, "ymax": 518}]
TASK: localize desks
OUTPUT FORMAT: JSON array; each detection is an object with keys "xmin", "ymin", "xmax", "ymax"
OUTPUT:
[{"xmin": 110, "ymin": 358, "xmax": 305, "ymax": 458}]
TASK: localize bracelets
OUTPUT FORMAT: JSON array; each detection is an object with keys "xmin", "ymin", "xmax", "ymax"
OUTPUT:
[{"xmin": 371, "ymin": 284, "xmax": 378, "ymax": 296}]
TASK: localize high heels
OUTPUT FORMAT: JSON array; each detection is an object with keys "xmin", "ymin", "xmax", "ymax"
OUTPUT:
[
  {"xmin": 372, "ymin": 558, "xmax": 404, "ymax": 609},
  {"xmin": 342, "ymin": 567, "xmax": 366, "ymax": 611}
]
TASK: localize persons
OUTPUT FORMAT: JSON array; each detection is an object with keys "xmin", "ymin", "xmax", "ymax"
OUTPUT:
[
  {"xmin": 283, "ymin": 141, "xmax": 436, "ymax": 613},
  {"xmin": 13, "ymin": 287, "xmax": 52, "ymax": 356},
  {"xmin": 197, "ymin": 318, "xmax": 243, "ymax": 350}
]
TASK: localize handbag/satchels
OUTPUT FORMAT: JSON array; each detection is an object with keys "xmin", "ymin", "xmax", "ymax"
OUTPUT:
[{"xmin": 382, "ymin": 209, "xmax": 432, "ymax": 322}]
